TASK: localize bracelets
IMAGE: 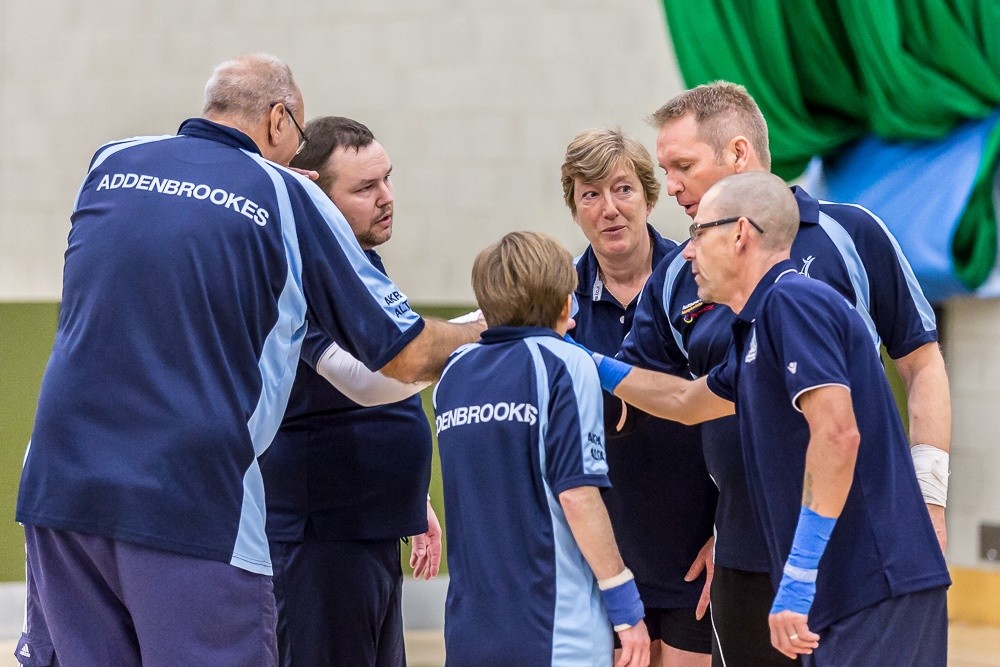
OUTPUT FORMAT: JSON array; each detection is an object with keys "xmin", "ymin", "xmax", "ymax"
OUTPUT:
[{"xmin": 910, "ymin": 443, "xmax": 950, "ymax": 508}]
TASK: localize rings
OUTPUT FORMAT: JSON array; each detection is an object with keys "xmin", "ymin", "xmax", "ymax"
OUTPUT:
[{"xmin": 790, "ymin": 634, "xmax": 798, "ymax": 639}]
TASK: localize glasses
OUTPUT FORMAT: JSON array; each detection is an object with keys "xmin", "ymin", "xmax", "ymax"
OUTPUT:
[
  {"xmin": 271, "ymin": 102, "xmax": 309, "ymax": 155},
  {"xmin": 688, "ymin": 215, "xmax": 764, "ymax": 238}
]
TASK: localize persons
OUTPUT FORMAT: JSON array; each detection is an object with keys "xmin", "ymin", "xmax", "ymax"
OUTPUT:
[
  {"xmin": 559, "ymin": 78, "xmax": 952, "ymax": 667},
  {"xmin": 565, "ymin": 170, "xmax": 952, "ymax": 667},
  {"xmin": 256, "ymin": 115, "xmax": 484, "ymax": 667},
  {"xmin": 433, "ymin": 231, "xmax": 653, "ymax": 667},
  {"xmin": 15, "ymin": 52, "xmax": 491, "ymax": 667}
]
